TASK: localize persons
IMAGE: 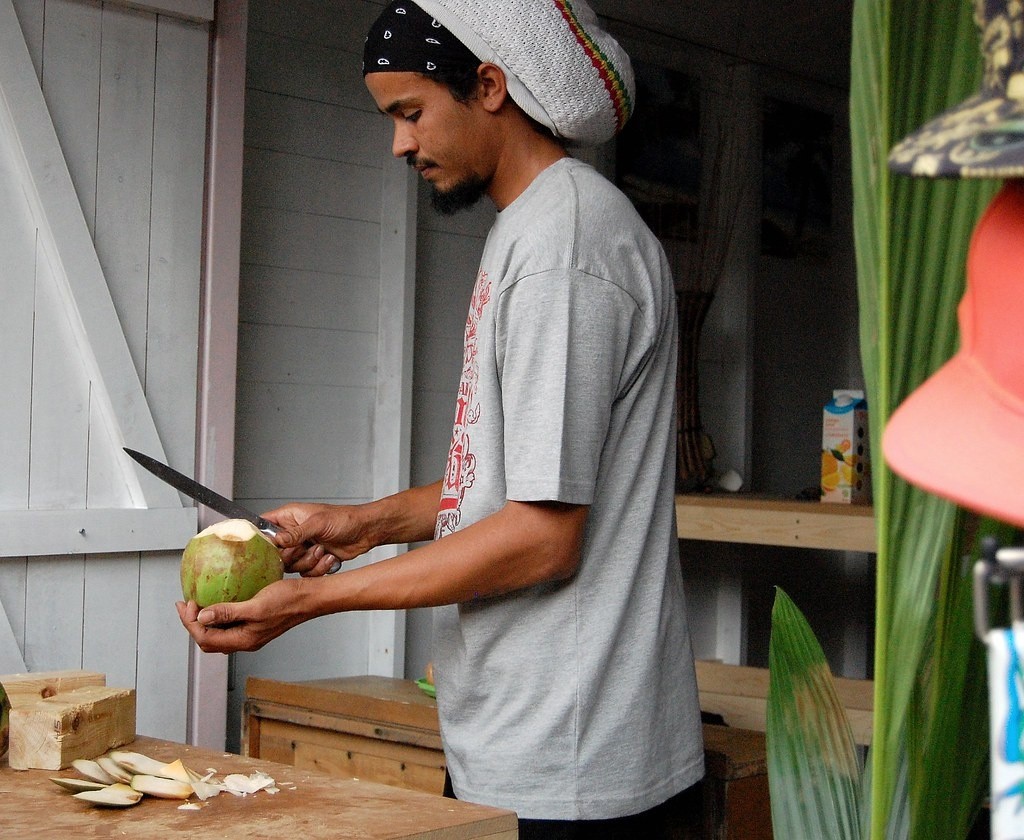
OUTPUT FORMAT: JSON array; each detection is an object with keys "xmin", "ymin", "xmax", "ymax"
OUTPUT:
[{"xmin": 172, "ymin": 0, "xmax": 705, "ymax": 840}]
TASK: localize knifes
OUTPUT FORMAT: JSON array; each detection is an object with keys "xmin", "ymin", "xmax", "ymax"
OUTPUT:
[{"xmin": 122, "ymin": 446, "xmax": 341, "ymax": 575}]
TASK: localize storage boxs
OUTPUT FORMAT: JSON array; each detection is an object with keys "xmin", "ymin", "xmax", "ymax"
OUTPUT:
[{"xmin": 820, "ymin": 388, "xmax": 873, "ymax": 505}]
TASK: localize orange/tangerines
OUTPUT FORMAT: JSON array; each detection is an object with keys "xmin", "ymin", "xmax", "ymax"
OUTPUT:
[{"xmin": 822, "ymin": 445, "xmax": 858, "ymax": 490}]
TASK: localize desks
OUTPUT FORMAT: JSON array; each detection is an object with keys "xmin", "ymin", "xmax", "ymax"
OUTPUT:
[{"xmin": 239, "ymin": 675, "xmax": 776, "ymax": 840}]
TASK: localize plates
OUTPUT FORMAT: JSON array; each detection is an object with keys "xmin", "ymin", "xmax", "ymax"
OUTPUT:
[{"xmin": 415, "ymin": 676, "xmax": 436, "ymax": 698}]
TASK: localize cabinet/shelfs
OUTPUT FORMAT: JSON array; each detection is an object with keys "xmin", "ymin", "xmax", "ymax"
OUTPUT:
[{"xmin": 674, "ymin": 495, "xmax": 875, "ymax": 745}]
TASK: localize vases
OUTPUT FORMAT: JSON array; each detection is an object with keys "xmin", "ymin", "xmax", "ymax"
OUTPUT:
[{"xmin": 675, "ymin": 290, "xmax": 715, "ymax": 494}]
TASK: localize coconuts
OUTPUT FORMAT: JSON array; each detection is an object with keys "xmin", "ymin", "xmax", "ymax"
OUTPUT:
[{"xmin": 181, "ymin": 518, "xmax": 285, "ymax": 609}]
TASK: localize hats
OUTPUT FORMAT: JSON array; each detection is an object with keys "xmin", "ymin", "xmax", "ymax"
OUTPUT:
[
  {"xmin": 887, "ymin": 1, "xmax": 1024, "ymax": 180},
  {"xmin": 411, "ymin": 0, "xmax": 636, "ymax": 149},
  {"xmin": 882, "ymin": 180, "xmax": 1024, "ymax": 529}
]
